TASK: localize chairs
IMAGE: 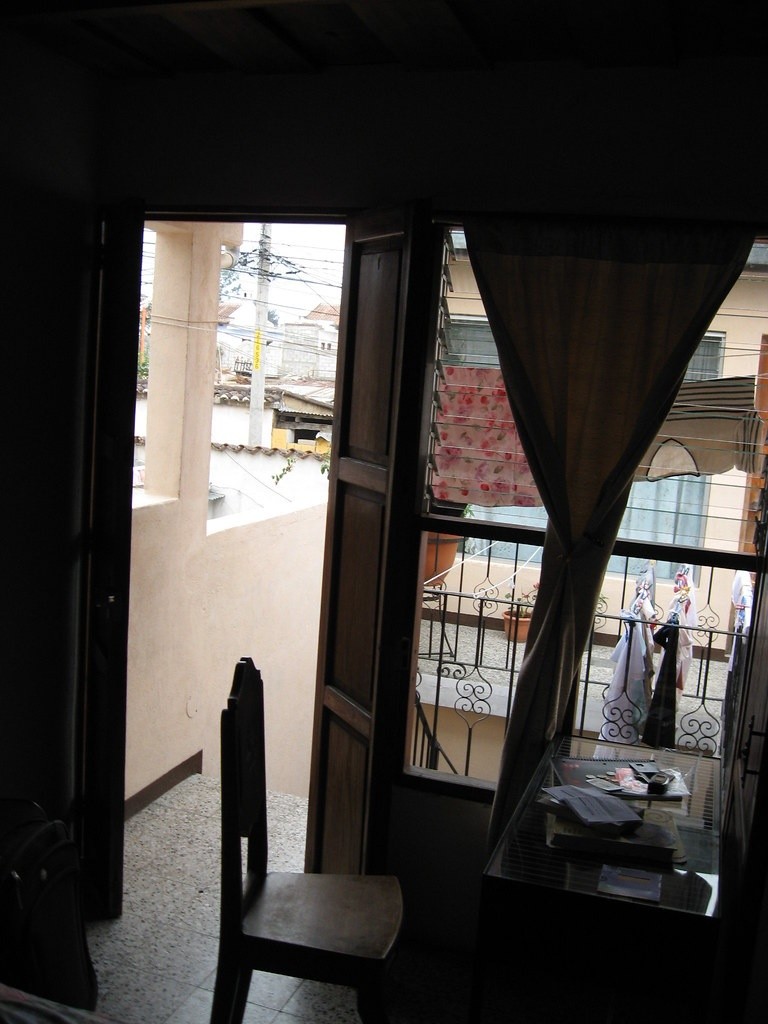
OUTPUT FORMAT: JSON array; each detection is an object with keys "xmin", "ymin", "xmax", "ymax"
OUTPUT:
[{"xmin": 211, "ymin": 655, "xmax": 406, "ymax": 1024}]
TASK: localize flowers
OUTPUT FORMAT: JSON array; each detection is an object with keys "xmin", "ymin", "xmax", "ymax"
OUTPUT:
[{"xmin": 504, "ymin": 583, "xmax": 539, "ymax": 618}]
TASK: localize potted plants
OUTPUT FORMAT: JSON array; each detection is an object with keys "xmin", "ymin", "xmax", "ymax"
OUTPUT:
[{"xmin": 423, "ymin": 504, "xmax": 475, "ymax": 586}]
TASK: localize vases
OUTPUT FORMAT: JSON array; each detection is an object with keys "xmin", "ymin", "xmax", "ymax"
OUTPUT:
[{"xmin": 502, "ymin": 611, "xmax": 532, "ymax": 643}]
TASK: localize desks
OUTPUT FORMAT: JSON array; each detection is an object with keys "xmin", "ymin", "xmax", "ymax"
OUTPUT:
[{"xmin": 466, "ymin": 732, "xmax": 722, "ymax": 1024}]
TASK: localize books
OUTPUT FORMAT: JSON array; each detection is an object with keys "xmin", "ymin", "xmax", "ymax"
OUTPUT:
[{"xmin": 541, "ymin": 785, "xmax": 644, "ymax": 826}]
